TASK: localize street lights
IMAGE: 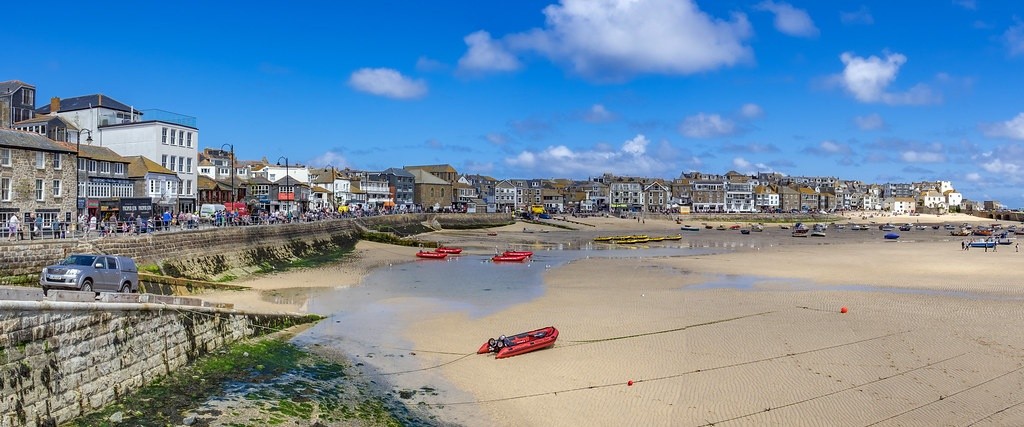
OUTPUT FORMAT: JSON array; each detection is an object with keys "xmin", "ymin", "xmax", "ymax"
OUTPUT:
[
  {"xmin": 218, "ymin": 143, "xmax": 234, "ymax": 210},
  {"xmin": 74, "ymin": 127, "xmax": 93, "ymax": 230},
  {"xmin": 323, "ymin": 164, "xmax": 336, "ymax": 212},
  {"xmin": 276, "ymin": 156, "xmax": 290, "ymax": 212}
]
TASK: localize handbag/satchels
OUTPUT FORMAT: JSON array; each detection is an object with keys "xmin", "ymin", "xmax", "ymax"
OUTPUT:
[{"xmin": 34, "ymin": 226, "xmax": 38, "ymax": 234}]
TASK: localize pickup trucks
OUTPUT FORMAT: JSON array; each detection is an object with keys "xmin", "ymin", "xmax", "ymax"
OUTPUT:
[{"xmin": 40, "ymin": 254, "xmax": 139, "ymax": 300}]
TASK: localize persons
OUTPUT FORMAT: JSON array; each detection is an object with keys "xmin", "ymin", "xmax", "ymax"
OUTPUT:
[
  {"xmin": 1016, "ymin": 243, "xmax": 1018, "ymax": 252},
  {"xmin": 962, "ymin": 240, "xmax": 997, "ymax": 252},
  {"xmin": 7, "ymin": 199, "xmax": 468, "ymax": 242}
]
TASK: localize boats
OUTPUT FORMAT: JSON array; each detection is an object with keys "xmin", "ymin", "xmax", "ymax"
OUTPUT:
[
  {"xmin": 476, "ymin": 326, "xmax": 559, "ymax": 360},
  {"xmin": 414, "ymin": 219, "xmax": 1024, "ymax": 263}
]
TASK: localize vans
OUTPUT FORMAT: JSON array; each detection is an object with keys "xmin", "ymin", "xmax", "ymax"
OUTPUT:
[{"xmin": 200, "ymin": 203, "xmax": 228, "ymax": 223}]
